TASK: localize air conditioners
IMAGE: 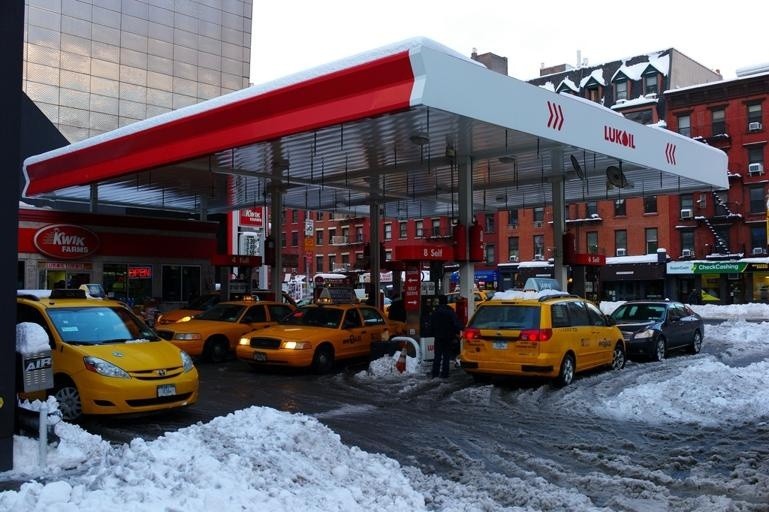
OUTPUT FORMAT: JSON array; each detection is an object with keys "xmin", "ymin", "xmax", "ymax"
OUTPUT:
[
  {"xmin": 748, "ymin": 121, "xmax": 762, "ymax": 131},
  {"xmin": 748, "ymin": 162, "xmax": 763, "ymax": 176},
  {"xmin": 681, "ymin": 209, "xmax": 693, "ymax": 219},
  {"xmin": 753, "ymin": 247, "xmax": 763, "ymax": 254},
  {"xmin": 616, "ymin": 247, "xmax": 626, "ymax": 256},
  {"xmin": 534, "ymin": 253, "xmax": 542, "ymax": 261},
  {"xmin": 509, "ymin": 254, "xmax": 517, "ymax": 261},
  {"xmin": 682, "ymin": 249, "xmax": 690, "ymax": 256}
]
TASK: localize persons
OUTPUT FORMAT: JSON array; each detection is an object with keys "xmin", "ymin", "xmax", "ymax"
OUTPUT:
[
  {"xmin": 430, "ymin": 295, "xmax": 464, "ymax": 378},
  {"xmin": 313, "ymin": 276, "xmax": 330, "ymax": 299},
  {"xmin": 389, "ymin": 294, "xmax": 407, "ymax": 323}
]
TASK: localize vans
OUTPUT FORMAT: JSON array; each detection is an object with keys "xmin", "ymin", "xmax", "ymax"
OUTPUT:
[
  {"xmin": 458, "ymin": 293, "xmax": 627, "ymax": 385},
  {"xmin": 16, "ymin": 287, "xmax": 200, "ymax": 424}
]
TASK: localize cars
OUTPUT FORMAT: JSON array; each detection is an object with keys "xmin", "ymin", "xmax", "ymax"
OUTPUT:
[
  {"xmin": 153, "ymin": 287, "xmax": 405, "ymax": 374},
  {"xmin": 443, "ymin": 291, "xmax": 488, "ymax": 323},
  {"xmin": 609, "ymin": 301, "xmax": 705, "ymax": 362}
]
